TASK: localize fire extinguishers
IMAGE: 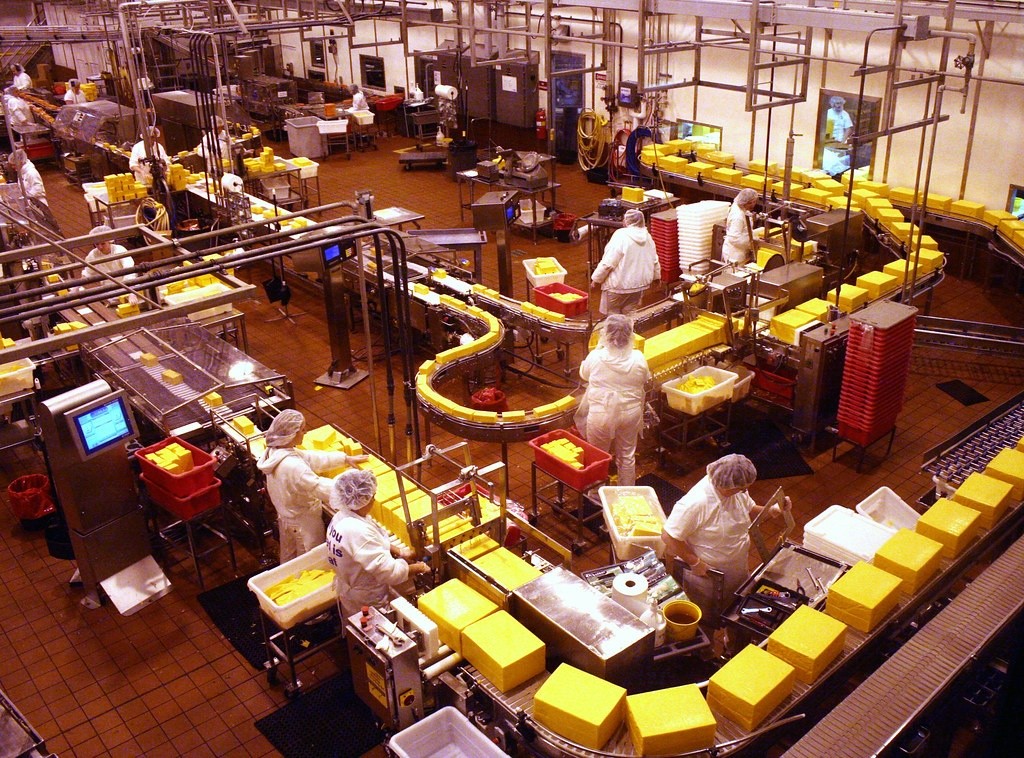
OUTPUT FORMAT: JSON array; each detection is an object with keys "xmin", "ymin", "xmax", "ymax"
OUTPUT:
[{"xmin": 535, "ymin": 107, "xmax": 548, "ymax": 140}]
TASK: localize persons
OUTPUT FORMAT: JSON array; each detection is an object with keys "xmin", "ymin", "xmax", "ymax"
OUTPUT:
[
  {"xmin": 823, "ymin": 95, "xmax": 853, "ymax": 176},
  {"xmin": 8, "ymin": 148, "xmax": 49, "ymax": 221},
  {"xmin": 721, "ymin": 187, "xmax": 765, "ymax": 273},
  {"xmin": 661, "ymin": 453, "xmax": 792, "ymax": 666},
  {"xmin": 573, "ymin": 313, "xmax": 650, "ymax": 502},
  {"xmin": 591, "ymin": 209, "xmax": 662, "ymax": 318},
  {"xmin": 197, "ymin": 115, "xmax": 232, "ymax": 177},
  {"xmin": 8, "ymin": 84, "xmax": 39, "ymax": 142},
  {"xmin": 69, "ymin": 225, "xmax": 138, "ymax": 307},
  {"xmin": 257, "ymin": 408, "xmax": 370, "ymax": 565},
  {"xmin": 4, "ymin": 63, "xmax": 32, "ymax": 93},
  {"xmin": 129, "ymin": 125, "xmax": 172, "ymax": 186},
  {"xmin": 64, "ymin": 78, "xmax": 86, "ymax": 105},
  {"xmin": 327, "ymin": 469, "xmax": 430, "ymax": 638},
  {"xmin": 346, "ymin": 84, "xmax": 369, "ymax": 136}
]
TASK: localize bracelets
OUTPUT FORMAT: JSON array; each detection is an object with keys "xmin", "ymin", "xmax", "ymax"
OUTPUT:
[{"xmin": 689, "ymin": 558, "xmax": 701, "ymax": 567}]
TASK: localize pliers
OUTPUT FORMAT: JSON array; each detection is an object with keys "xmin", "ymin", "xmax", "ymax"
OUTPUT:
[{"xmin": 796, "ymin": 578, "xmax": 805, "ymax": 595}]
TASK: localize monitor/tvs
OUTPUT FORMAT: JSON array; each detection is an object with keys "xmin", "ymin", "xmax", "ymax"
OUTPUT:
[{"xmin": 64, "ymin": 389, "xmax": 140, "ymax": 462}]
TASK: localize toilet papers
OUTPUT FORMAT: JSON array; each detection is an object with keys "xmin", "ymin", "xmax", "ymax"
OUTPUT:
[
  {"xmin": 612, "ymin": 573, "xmax": 649, "ymax": 618},
  {"xmin": 221, "ymin": 173, "xmax": 243, "ymax": 192},
  {"xmin": 415, "ymin": 91, "xmax": 424, "ymax": 100},
  {"xmin": 434, "ymin": 84, "xmax": 458, "ymax": 100},
  {"xmin": 571, "ymin": 224, "xmax": 598, "ymax": 241}
]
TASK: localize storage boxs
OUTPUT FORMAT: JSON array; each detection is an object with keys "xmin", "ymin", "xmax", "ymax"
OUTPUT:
[
  {"xmin": 532, "ymin": 282, "xmax": 589, "ymax": 318},
  {"xmin": 134, "ymin": 435, "xmax": 222, "ymax": 521},
  {"xmin": 802, "ymin": 485, "xmax": 923, "ymax": 566},
  {"xmin": 388, "ymin": 707, "xmax": 513, "ymax": 758},
  {"xmin": 742, "ymin": 363, "xmax": 800, "ymax": 399},
  {"xmin": 163, "ymin": 282, "xmax": 233, "ymax": 322},
  {"xmin": 522, "ymin": 257, "xmax": 568, "ymax": 287},
  {"xmin": 284, "ymin": 116, "xmax": 328, "ymax": 159},
  {"xmin": 598, "ymin": 486, "xmax": 667, "ymax": 560},
  {"xmin": 316, "ymin": 119, "xmax": 348, "ymax": 133},
  {"xmin": 712, "ymin": 361, "xmax": 755, "ymax": 403},
  {"xmin": 374, "ymin": 96, "xmax": 404, "ymax": 111},
  {"xmin": 248, "ymin": 539, "xmax": 341, "ymax": 631},
  {"xmin": 650, "ymin": 200, "xmax": 732, "ymax": 286},
  {"xmin": 528, "ymin": 429, "xmax": 612, "ymax": 492},
  {"xmin": 83, "ymin": 181, "xmax": 141, "ymax": 229},
  {"xmin": 0, "ymin": 358, "xmax": 36, "ymax": 397},
  {"xmin": 260, "ymin": 178, "xmax": 290, "ymax": 200},
  {"xmin": 516, "ymin": 198, "xmax": 546, "ymax": 224},
  {"xmin": 837, "ymin": 300, "xmax": 919, "ymax": 448},
  {"xmin": 15, "ymin": 138, "xmax": 55, "ymax": 161},
  {"xmin": 661, "ymin": 365, "xmax": 738, "ymax": 416},
  {"xmin": 352, "ymin": 110, "xmax": 375, "ymax": 125},
  {"xmin": 285, "ymin": 156, "xmax": 319, "ymax": 179}
]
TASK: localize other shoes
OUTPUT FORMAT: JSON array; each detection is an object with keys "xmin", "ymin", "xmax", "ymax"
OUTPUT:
[
  {"xmin": 305, "ymin": 612, "xmax": 330, "ymax": 626},
  {"xmin": 587, "ymin": 488, "xmax": 602, "ymax": 507}
]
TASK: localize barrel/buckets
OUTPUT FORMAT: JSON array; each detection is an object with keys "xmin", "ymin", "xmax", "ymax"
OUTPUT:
[{"xmin": 663, "ymin": 600, "xmax": 702, "ymax": 642}]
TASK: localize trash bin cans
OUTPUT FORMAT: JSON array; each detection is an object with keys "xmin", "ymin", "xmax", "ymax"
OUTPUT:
[
  {"xmin": 8, "ymin": 474, "xmax": 51, "ymax": 534},
  {"xmin": 176, "ymin": 218, "xmax": 214, "ymax": 253}
]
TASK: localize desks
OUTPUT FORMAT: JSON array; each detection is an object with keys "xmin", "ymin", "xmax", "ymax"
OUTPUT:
[
  {"xmin": 194, "ymin": 307, "xmax": 250, "ymax": 357},
  {"xmin": 372, "ymin": 205, "xmax": 426, "ymax": 231},
  {"xmin": 259, "ymin": 606, "xmax": 343, "ymax": 697},
  {"xmin": 530, "ymin": 460, "xmax": 611, "ymax": 557},
  {"xmin": 832, "ymin": 425, "xmax": 896, "ymax": 474},
  {"xmin": 654, "ymin": 396, "xmax": 733, "ymax": 476},
  {"xmin": 0, "ymin": 389, "xmax": 39, "ymax": 451},
  {"xmin": 457, "ymin": 169, "xmax": 561, "ymax": 243},
  {"xmin": 144, "ymin": 496, "xmax": 236, "ymax": 591}
]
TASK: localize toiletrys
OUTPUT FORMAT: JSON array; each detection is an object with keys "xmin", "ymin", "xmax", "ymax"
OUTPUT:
[
  {"xmin": 414, "ymin": 83, "xmax": 421, "ymax": 98},
  {"xmin": 639, "ymin": 591, "xmax": 667, "ymax": 648},
  {"xmin": 436, "ymin": 126, "xmax": 444, "ymax": 146}
]
